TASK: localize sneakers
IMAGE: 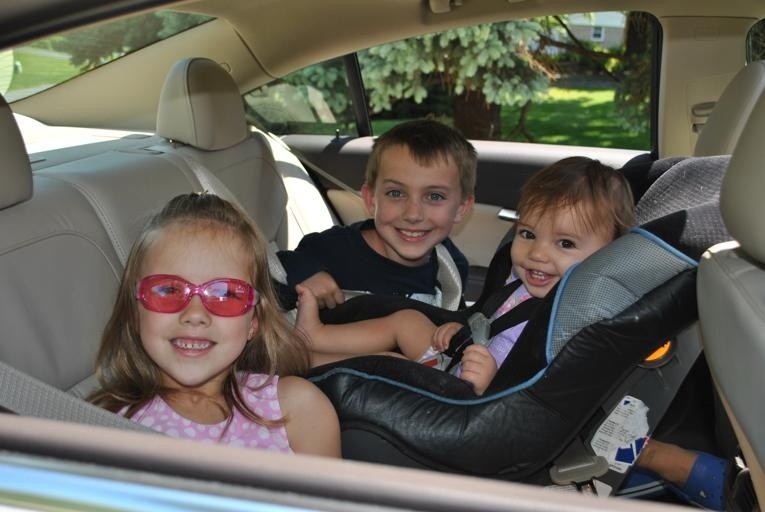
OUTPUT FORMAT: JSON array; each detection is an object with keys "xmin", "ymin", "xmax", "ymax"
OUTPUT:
[
  {"xmin": 667, "ymin": 452, "xmax": 741, "ymax": 512},
  {"xmin": 615, "ymin": 468, "xmax": 668, "ymax": 498}
]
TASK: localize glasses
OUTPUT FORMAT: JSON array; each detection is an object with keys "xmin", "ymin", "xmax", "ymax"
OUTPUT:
[{"xmin": 136, "ymin": 274, "xmax": 261, "ymax": 317}]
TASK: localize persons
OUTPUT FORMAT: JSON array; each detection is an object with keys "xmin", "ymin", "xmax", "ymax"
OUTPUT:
[
  {"xmin": 268, "ymin": 117, "xmax": 470, "ymax": 309},
  {"xmin": 617, "ymin": 429, "xmax": 726, "ymax": 511},
  {"xmin": 74, "ymin": 188, "xmax": 341, "ymax": 460},
  {"xmin": 297, "ymin": 155, "xmax": 638, "ymax": 397}
]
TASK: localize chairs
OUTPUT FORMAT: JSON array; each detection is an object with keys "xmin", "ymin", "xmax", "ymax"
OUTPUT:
[
  {"xmin": 690, "ymin": 58, "xmax": 765, "ymax": 507},
  {"xmin": 0, "ymin": 90, "xmax": 166, "ymax": 443},
  {"xmin": 152, "ymin": 58, "xmax": 325, "ymax": 314},
  {"xmin": 297, "ymin": 153, "xmax": 735, "ymax": 496}
]
